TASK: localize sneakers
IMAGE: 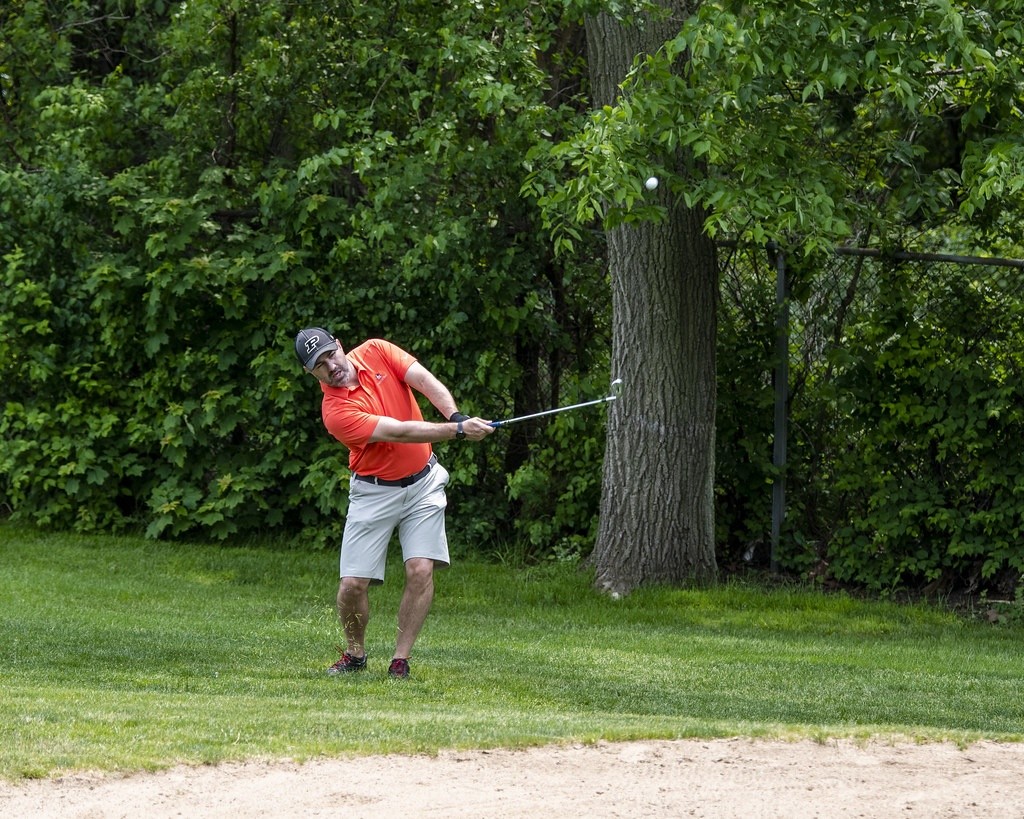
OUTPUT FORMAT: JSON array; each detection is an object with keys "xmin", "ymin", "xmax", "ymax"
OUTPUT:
[
  {"xmin": 388, "ymin": 659, "xmax": 408, "ymax": 677},
  {"xmin": 329, "ymin": 651, "xmax": 367, "ymax": 674}
]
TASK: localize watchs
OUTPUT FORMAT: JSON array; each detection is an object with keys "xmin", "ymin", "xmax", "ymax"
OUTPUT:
[{"xmin": 456, "ymin": 422, "xmax": 466, "ymax": 439}]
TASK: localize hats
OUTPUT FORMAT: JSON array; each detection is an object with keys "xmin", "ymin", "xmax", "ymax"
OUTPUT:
[{"xmin": 295, "ymin": 327, "xmax": 338, "ymax": 371}]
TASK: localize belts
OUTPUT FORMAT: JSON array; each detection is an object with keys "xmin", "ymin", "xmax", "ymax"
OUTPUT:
[{"xmin": 352, "ymin": 455, "xmax": 436, "ymax": 488}]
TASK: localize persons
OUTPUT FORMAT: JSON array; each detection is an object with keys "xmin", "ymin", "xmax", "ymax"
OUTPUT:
[{"xmin": 295, "ymin": 327, "xmax": 496, "ymax": 679}]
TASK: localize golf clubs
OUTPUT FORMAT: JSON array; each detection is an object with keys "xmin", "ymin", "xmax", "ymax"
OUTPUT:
[{"xmin": 481, "ymin": 377, "xmax": 625, "ymax": 428}]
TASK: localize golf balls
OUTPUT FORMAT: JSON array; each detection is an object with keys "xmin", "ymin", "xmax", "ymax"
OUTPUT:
[{"xmin": 645, "ymin": 177, "xmax": 659, "ymax": 190}]
